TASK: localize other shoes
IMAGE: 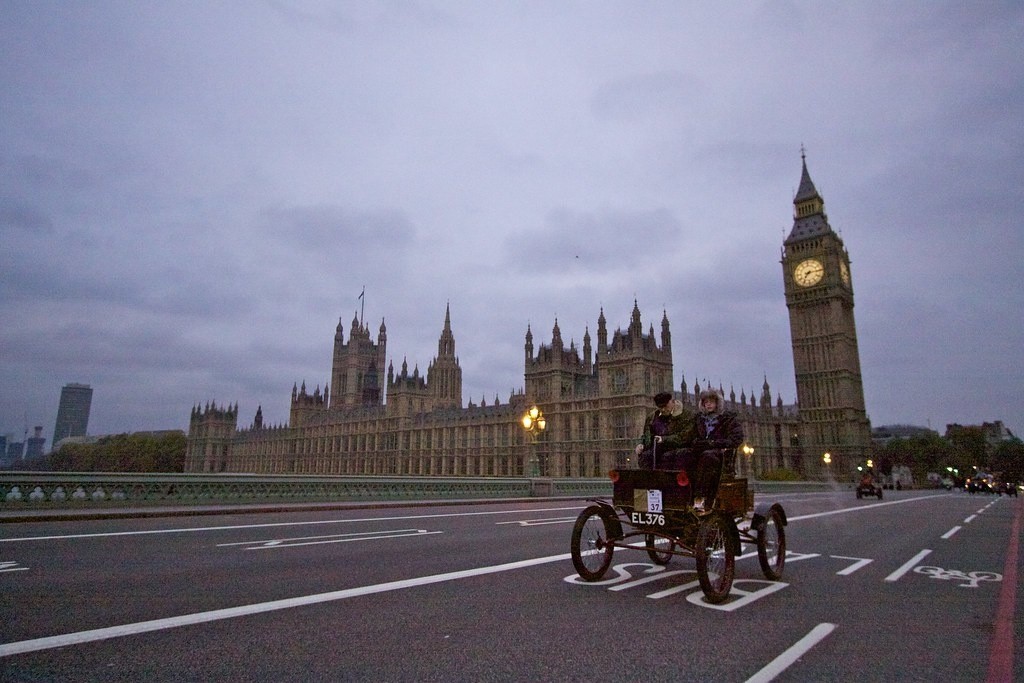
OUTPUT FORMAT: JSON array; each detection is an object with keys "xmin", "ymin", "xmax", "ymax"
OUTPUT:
[{"xmin": 691, "ymin": 496, "xmax": 706, "ymax": 513}]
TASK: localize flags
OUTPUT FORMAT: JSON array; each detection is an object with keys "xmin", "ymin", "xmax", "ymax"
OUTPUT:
[{"xmin": 358, "ymin": 291, "xmax": 363, "ymax": 299}]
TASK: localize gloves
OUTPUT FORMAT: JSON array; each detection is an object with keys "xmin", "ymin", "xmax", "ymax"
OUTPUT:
[
  {"xmin": 635, "ymin": 444, "xmax": 645, "ymax": 455},
  {"xmin": 654, "ymin": 435, "xmax": 665, "ymax": 444}
]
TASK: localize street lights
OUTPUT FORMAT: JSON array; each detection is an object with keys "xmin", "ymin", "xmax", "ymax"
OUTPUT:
[
  {"xmin": 522, "ymin": 404, "xmax": 546, "ymax": 473},
  {"xmin": 824, "ymin": 454, "xmax": 831, "ymax": 480},
  {"xmin": 743, "ymin": 444, "xmax": 755, "ymax": 474}
]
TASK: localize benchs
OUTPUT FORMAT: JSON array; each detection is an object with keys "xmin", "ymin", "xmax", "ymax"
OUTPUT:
[{"xmin": 704, "ymin": 447, "xmax": 738, "ymax": 509}]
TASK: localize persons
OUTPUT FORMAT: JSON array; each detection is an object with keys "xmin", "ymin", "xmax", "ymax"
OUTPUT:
[
  {"xmin": 676, "ymin": 389, "xmax": 744, "ymax": 512},
  {"xmin": 635, "ymin": 392, "xmax": 694, "ymax": 469},
  {"xmin": 861, "ymin": 471, "xmax": 872, "ymax": 484}
]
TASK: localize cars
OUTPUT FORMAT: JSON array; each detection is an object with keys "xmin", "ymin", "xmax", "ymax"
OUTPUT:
[
  {"xmin": 570, "ymin": 439, "xmax": 787, "ymax": 601},
  {"xmin": 856, "ymin": 478, "xmax": 882, "ymax": 500}
]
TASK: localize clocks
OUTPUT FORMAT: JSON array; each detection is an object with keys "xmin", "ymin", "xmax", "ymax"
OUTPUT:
[
  {"xmin": 793, "ymin": 258, "xmax": 825, "ymax": 287},
  {"xmin": 839, "ymin": 256, "xmax": 851, "ymax": 287}
]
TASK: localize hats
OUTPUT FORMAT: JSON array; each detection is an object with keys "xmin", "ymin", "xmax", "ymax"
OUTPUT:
[
  {"xmin": 700, "ymin": 389, "xmax": 724, "ymax": 415},
  {"xmin": 653, "ymin": 392, "xmax": 673, "ymax": 409}
]
